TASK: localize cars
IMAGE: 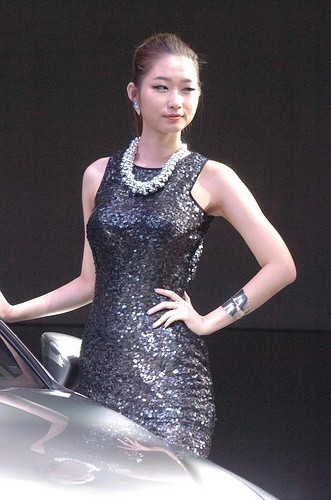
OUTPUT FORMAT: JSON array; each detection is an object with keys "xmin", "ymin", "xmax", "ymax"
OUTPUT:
[{"xmin": 0, "ymin": 316, "xmax": 285, "ymax": 500}]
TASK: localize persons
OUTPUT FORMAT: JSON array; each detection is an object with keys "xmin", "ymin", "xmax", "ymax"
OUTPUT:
[
  {"xmin": 0, "ymin": 32, "xmax": 297, "ymax": 461},
  {"xmin": 0, "ymin": 390, "xmax": 203, "ymax": 490}
]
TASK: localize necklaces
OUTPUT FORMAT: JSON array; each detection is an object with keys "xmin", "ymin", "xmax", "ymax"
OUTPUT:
[
  {"xmin": 56, "ymin": 457, "xmax": 101, "ymax": 473},
  {"xmin": 120, "ymin": 134, "xmax": 188, "ymax": 195}
]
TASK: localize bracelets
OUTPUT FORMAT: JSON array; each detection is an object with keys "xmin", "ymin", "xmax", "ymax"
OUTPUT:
[
  {"xmin": 222, "ymin": 290, "xmax": 251, "ymax": 320},
  {"xmin": 177, "ymin": 460, "xmax": 189, "ymax": 471}
]
